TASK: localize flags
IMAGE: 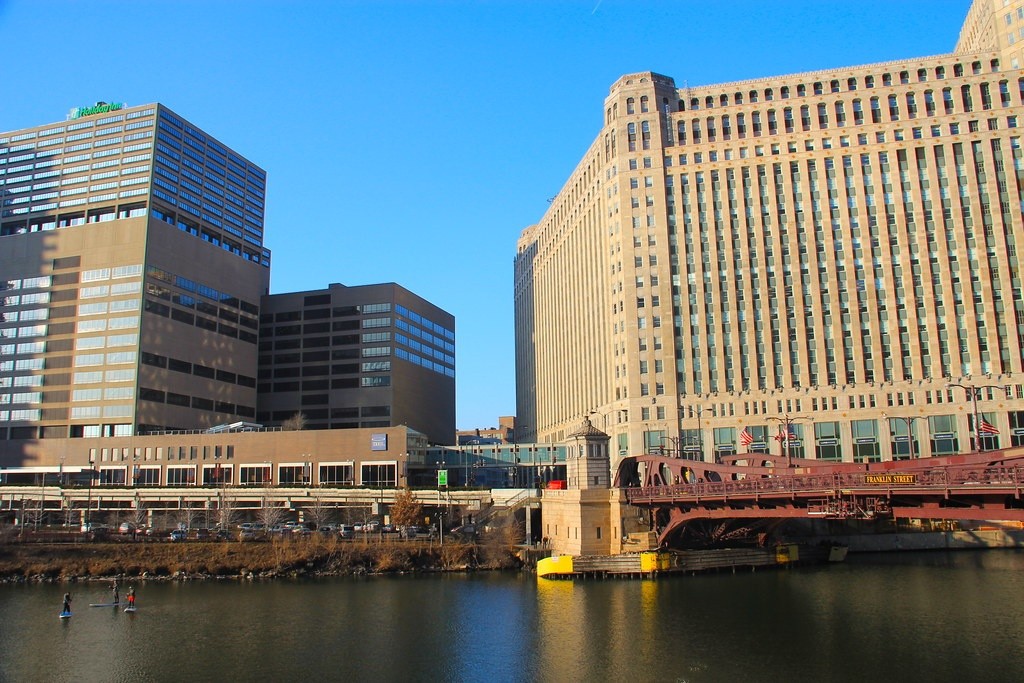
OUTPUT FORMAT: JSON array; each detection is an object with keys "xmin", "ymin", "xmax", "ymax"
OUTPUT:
[
  {"xmin": 782, "ymin": 423, "xmax": 794, "ymax": 440},
  {"xmin": 740, "ymin": 428, "xmax": 753, "ymax": 444},
  {"xmin": 980, "ymin": 413, "xmax": 1000, "ymax": 434}
]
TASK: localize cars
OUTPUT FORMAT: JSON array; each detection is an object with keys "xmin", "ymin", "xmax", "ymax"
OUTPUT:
[
  {"xmin": 170, "ymin": 530, "xmax": 187, "ymax": 540},
  {"xmin": 81, "ymin": 523, "xmax": 110, "ymax": 531},
  {"xmin": 237, "ymin": 521, "xmax": 309, "ymax": 533},
  {"xmin": 196, "ymin": 528, "xmax": 212, "ymax": 538},
  {"xmin": 239, "ymin": 530, "xmax": 255, "ymax": 539},
  {"xmin": 319, "ymin": 521, "xmax": 421, "ymax": 538},
  {"xmin": 217, "ymin": 529, "xmax": 232, "ymax": 540},
  {"xmin": 118, "ymin": 522, "xmax": 135, "ymax": 534}
]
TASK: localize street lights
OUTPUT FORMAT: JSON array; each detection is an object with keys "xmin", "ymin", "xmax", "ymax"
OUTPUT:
[
  {"xmin": 883, "ymin": 416, "xmax": 929, "ymax": 459},
  {"xmin": 466, "ymin": 440, "xmax": 476, "ymax": 486},
  {"xmin": 764, "ymin": 417, "xmax": 814, "ymax": 467},
  {"xmin": 945, "ymin": 383, "xmax": 1007, "ymax": 452},
  {"xmin": 677, "ymin": 407, "xmax": 713, "ymax": 461}
]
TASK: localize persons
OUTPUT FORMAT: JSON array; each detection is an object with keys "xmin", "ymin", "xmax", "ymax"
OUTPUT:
[
  {"xmin": 542, "ymin": 536, "xmax": 548, "ymax": 549},
  {"xmin": 62, "ymin": 592, "xmax": 72, "ymax": 614},
  {"xmin": 125, "ymin": 587, "xmax": 135, "ymax": 609},
  {"xmin": 533, "ymin": 536, "xmax": 537, "ymax": 549},
  {"xmin": 108, "ymin": 580, "xmax": 119, "ymax": 604}
]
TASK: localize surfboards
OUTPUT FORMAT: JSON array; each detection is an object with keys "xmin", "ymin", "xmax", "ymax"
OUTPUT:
[
  {"xmin": 59, "ymin": 611, "xmax": 72, "ymax": 618},
  {"xmin": 89, "ymin": 602, "xmax": 120, "ymax": 606},
  {"xmin": 124, "ymin": 606, "xmax": 138, "ymax": 612}
]
